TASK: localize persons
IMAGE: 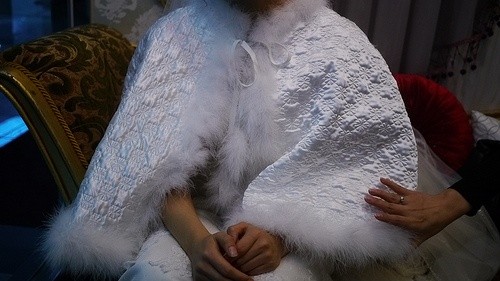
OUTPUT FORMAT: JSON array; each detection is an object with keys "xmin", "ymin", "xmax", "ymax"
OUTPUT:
[
  {"xmin": 47, "ymin": 0, "xmax": 419, "ymax": 281},
  {"xmin": 364, "ymin": 142, "xmax": 500, "ymax": 281}
]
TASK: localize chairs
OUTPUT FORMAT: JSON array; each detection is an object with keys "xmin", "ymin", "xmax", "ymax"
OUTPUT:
[{"xmin": 0, "ymin": 20, "xmax": 141, "ymax": 208}]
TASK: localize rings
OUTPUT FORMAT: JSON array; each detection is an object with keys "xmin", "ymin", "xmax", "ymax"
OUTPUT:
[{"xmin": 399, "ymin": 195, "xmax": 404, "ymax": 205}]
